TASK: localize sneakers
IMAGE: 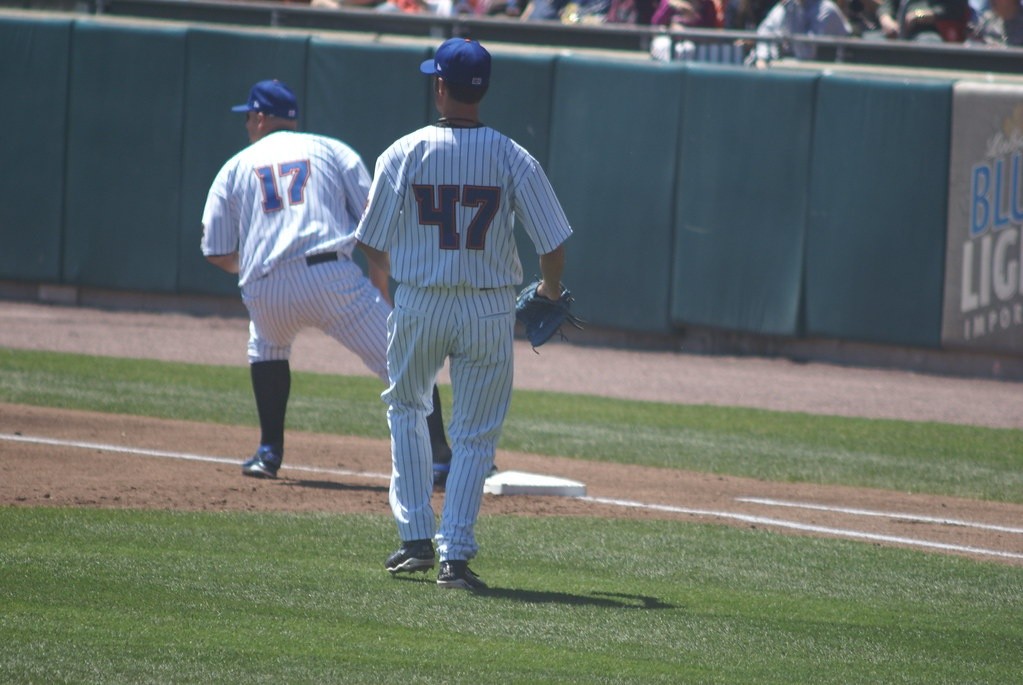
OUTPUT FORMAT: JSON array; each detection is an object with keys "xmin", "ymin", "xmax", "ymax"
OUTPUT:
[
  {"xmin": 242, "ymin": 446, "xmax": 281, "ymax": 478},
  {"xmin": 437, "ymin": 560, "xmax": 480, "ymax": 590},
  {"xmin": 384, "ymin": 541, "xmax": 435, "ymax": 573},
  {"xmin": 429, "ymin": 458, "xmax": 451, "ymax": 482}
]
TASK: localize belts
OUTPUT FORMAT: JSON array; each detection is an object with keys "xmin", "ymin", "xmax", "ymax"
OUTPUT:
[{"xmin": 264, "ymin": 251, "xmax": 338, "ymax": 278}]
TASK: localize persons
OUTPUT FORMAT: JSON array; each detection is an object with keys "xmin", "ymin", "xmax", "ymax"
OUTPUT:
[
  {"xmin": 310, "ymin": 1, "xmax": 1023, "ymax": 65},
  {"xmin": 201, "ymin": 79, "xmax": 452, "ymax": 481},
  {"xmin": 353, "ymin": 37, "xmax": 574, "ymax": 586}
]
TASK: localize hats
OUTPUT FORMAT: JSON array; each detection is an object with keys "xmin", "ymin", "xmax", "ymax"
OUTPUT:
[
  {"xmin": 231, "ymin": 79, "xmax": 297, "ymax": 120},
  {"xmin": 420, "ymin": 37, "xmax": 490, "ymax": 85}
]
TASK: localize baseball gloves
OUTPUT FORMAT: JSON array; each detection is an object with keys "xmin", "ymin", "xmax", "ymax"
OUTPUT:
[{"xmin": 516, "ymin": 278, "xmax": 573, "ymax": 347}]
